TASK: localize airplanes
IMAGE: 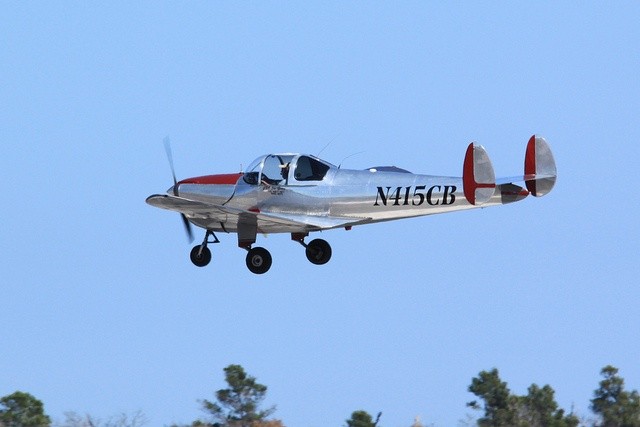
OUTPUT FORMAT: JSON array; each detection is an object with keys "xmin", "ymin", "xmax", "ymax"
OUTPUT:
[{"xmin": 147, "ymin": 133, "xmax": 558, "ymax": 275}]
